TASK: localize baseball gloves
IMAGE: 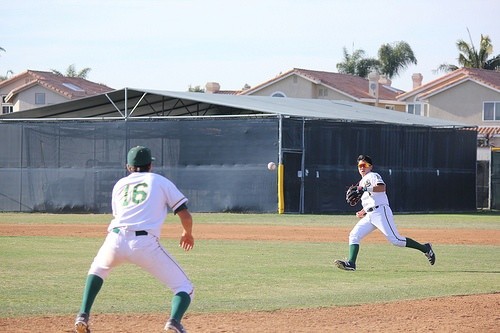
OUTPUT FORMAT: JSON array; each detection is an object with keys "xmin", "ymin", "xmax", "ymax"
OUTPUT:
[{"xmin": 345, "ymin": 186, "xmax": 367, "ymax": 206}]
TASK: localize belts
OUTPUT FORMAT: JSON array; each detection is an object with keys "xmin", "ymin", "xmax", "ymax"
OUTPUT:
[
  {"xmin": 113, "ymin": 227, "xmax": 149, "ymax": 236},
  {"xmin": 367, "ymin": 205, "xmax": 379, "ymax": 213}
]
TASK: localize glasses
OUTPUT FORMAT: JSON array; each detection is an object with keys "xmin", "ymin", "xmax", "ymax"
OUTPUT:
[{"xmin": 358, "ymin": 163, "xmax": 371, "ymax": 168}]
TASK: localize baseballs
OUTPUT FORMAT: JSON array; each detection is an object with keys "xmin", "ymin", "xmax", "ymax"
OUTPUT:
[{"xmin": 268, "ymin": 162, "xmax": 275, "ymax": 170}]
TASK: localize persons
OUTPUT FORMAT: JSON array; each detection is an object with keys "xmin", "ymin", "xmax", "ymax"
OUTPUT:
[
  {"xmin": 75, "ymin": 146, "xmax": 195, "ymax": 333},
  {"xmin": 334, "ymin": 154, "xmax": 436, "ymax": 271}
]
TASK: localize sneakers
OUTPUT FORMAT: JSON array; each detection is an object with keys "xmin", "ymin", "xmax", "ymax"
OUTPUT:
[
  {"xmin": 333, "ymin": 259, "xmax": 357, "ymax": 270},
  {"xmin": 73, "ymin": 312, "xmax": 90, "ymax": 333},
  {"xmin": 163, "ymin": 318, "xmax": 186, "ymax": 332},
  {"xmin": 423, "ymin": 241, "xmax": 435, "ymax": 267}
]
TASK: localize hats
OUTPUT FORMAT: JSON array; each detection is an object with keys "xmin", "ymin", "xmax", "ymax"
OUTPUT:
[{"xmin": 127, "ymin": 144, "xmax": 158, "ymax": 168}]
